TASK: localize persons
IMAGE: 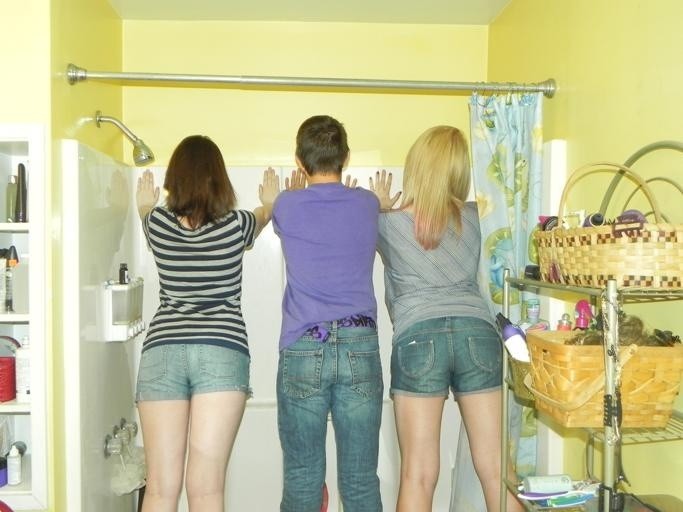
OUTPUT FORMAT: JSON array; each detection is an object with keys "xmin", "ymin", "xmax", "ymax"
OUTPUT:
[
  {"xmin": 367, "ymin": 124, "xmax": 529, "ymax": 512},
  {"xmin": 130, "ymin": 135, "xmax": 281, "ymax": 511},
  {"xmin": 268, "ymin": 114, "xmax": 386, "ymax": 512}
]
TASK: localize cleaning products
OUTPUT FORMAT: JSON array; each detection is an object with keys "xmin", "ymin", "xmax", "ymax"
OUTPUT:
[{"xmin": 573, "ymin": 299, "xmax": 593, "ymax": 330}]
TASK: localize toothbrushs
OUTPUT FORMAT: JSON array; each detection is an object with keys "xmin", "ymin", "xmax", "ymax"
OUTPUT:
[{"xmin": 517, "ymin": 483, "xmax": 601, "ymax": 507}]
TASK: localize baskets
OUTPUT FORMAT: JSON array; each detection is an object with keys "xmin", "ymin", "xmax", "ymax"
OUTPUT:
[
  {"xmin": 534, "ymin": 140, "xmax": 682, "ymax": 292},
  {"xmin": 523, "ymin": 330, "xmax": 682, "ymax": 429}
]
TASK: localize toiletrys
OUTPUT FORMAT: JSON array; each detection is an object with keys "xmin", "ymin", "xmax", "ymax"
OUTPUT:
[
  {"xmin": 5, "ymin": 175, "xmax": 17, "ymax": 222},
  {"xmin": 118, "ymin": 263, "xmax": 128, "ymax": 284}
]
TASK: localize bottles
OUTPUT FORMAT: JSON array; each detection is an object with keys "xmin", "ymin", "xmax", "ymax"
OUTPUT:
[
  {"xmin": 501, "ymin": 326, "xmax": 530, "ymax": 363},
  {"xmin": 526, "ymin": 298, "xmax": 541, "ymax": 319}
]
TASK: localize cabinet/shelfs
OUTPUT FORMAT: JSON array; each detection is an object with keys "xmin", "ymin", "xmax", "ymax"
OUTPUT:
[
  {"xmin": 0, "ymin": 119, "xmax": 47, "ymax": 512},
  {"xmin": 499, "ymin": 268, "xmax": 683, "ymax": 512}
]
